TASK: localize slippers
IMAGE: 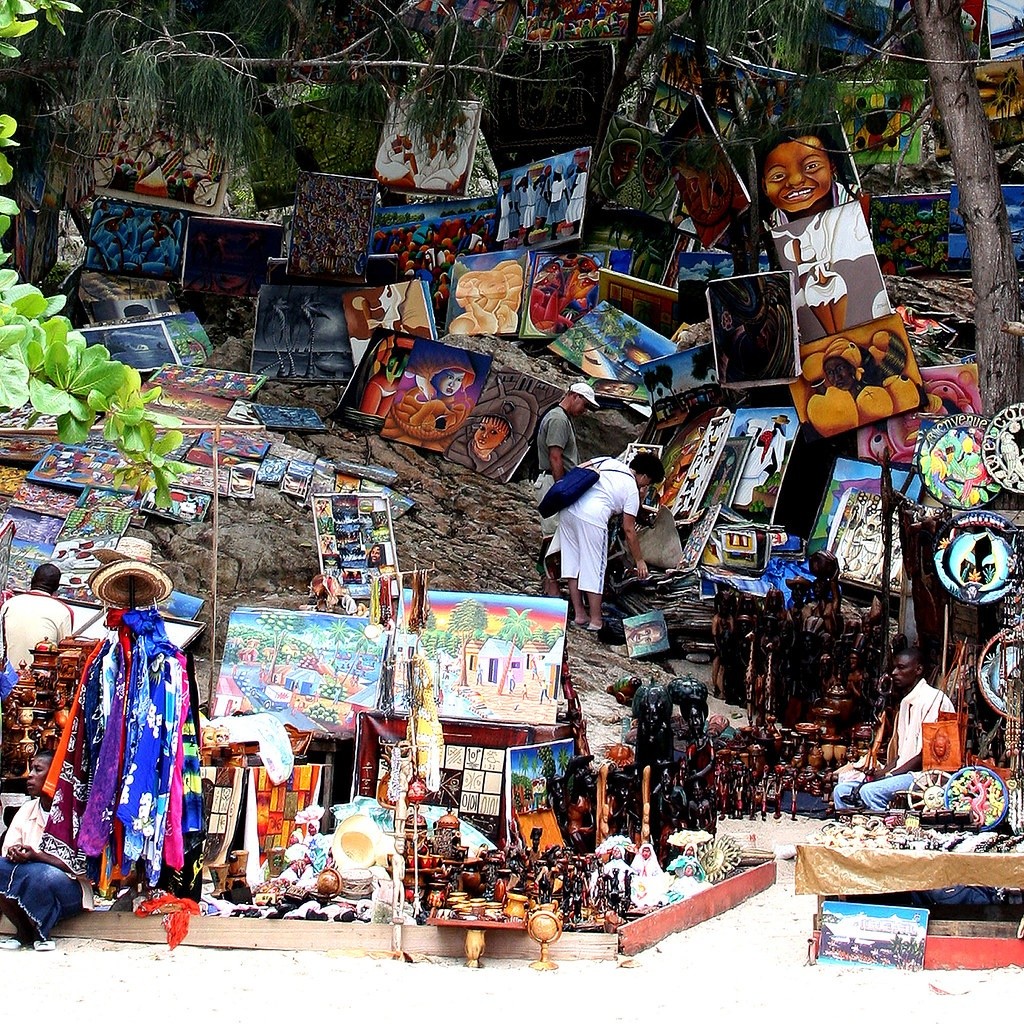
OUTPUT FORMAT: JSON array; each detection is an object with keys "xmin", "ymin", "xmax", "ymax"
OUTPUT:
[
  {"xmin": 586, "ymin": 619, "xmax": 608, "ymax": 632},
  {"xmin": 579, "ymin": 621, "xmax": 590, "ymax": 628}
]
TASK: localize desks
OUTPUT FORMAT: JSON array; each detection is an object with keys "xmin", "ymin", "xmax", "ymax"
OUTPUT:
[
  {"xmin": 795, "ymin": 842, "xmax": 1024, "ymax": 968},
  {"xmin": 348, "ymin": 712, "xmax": 572, "ymax": 849},
  {"xmin": 429, "ymin": 908, "xmax": 530, "ymax": 970},
  {"xmin": 133, "ymin": 763, "xmax": 331, "ymax": 903}
]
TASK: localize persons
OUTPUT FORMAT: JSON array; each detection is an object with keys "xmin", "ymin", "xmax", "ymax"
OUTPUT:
[
  {"xmin": 0, "ymin": 563, "xmax": 72, "ymax": 672},
  {"xmin": 0, "ymin": 750, "xmax": 94, "ymax": 952},
  {"xmin": 548, "ymin": 674, "xmax": 797, "ymax": 855},
  {"xmin": 475, "ymin": 829, "xmax": 714, "ymax": 933},
  {"xmin": 533, "ymin": 383, "xmax": 665, "ymax": 633},
  {"xmin": 711, "ymin": 551, "xmax": 909, "ymax": 726},
  {"xmin": 833, "ymin": 650, "xmax": 956, "ymax": 814}
]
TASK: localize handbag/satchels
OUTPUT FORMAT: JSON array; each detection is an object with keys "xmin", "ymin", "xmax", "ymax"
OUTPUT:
[
  {"xmin": 921, "ymin": 646, "xmax": 968, "ymax": 772},
  {"xmin": 537, "ymin": 458, "xmax": 634, "ymax": 520}
]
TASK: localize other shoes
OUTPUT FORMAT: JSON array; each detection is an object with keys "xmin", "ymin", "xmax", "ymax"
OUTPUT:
[
  {"xmin": 0, "ymin": 938, "xmax": 22, "ymax": 950},
  {"xmin": 34, "ymin": 940, "xmax": 56, "ymax": 950}
]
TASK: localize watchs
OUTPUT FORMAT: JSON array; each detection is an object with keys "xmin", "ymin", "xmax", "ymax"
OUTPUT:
[{"xmin": 885, "ymin": 772, "xmax": 893, "ymax": 778}]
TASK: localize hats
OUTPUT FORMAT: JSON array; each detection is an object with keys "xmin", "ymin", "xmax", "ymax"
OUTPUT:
[
  {"xmin": 92, "ymin": 537, "xmax": 155, "ymax": 566},
  {"xmin": 567, "ymin": 383, "xmax": 600, "ymax": 409},
  {"xmin": 87, "ymin": 559, "xmax": 174, "ymax": 609},
  {"xmin": 332, "ymin": 814, "xmax": 384, "ymax": 869}
]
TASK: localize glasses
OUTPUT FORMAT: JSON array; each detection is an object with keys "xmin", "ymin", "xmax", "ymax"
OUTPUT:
[{"xmin": 578, "ymin": 395, "xmax": 592, "ymax": 409}]
